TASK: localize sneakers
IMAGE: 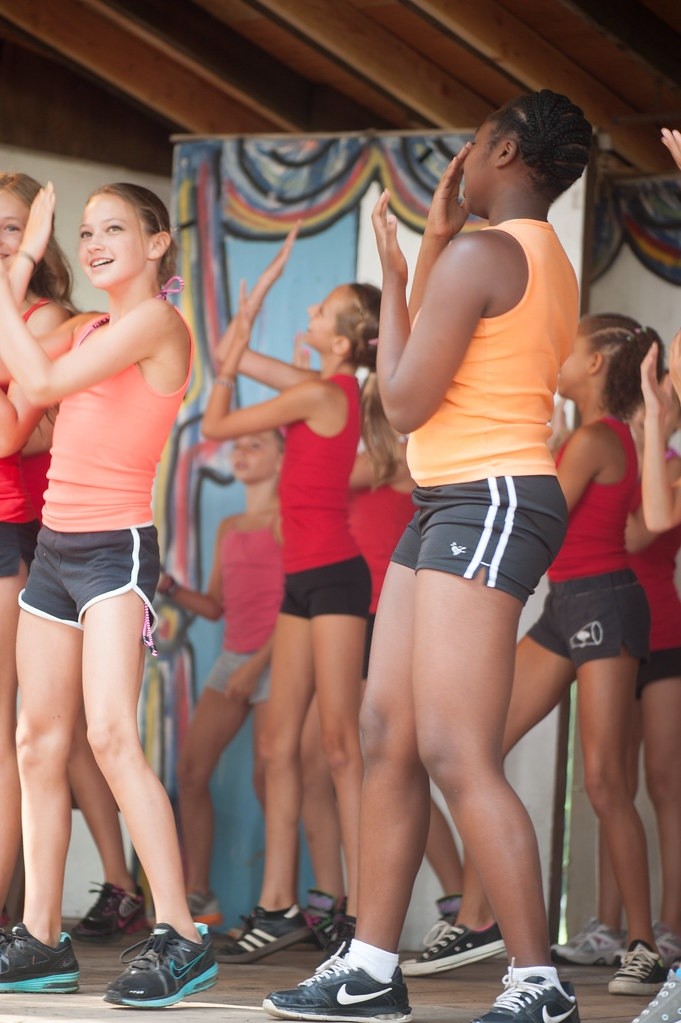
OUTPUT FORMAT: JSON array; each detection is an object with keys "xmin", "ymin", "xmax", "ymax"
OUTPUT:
[
  {"xmin": 472, "ymin": 957, "xmax": 581, "ymax": 1023},
  {"xmin": 0, "ymin": 922, "xmax": 80, "ymax": 992},
  {"xmin": 262, "ymin": 940, "xmax": 411, "ymax": 1023},
  {"xmin": 650, "ymin": 923, "xmax": 681, "ymax": 964},
  {"xmin": 549, "ymin": 918, "xmax": 627, "ymax": 966},
  {"xmin": 400, "ymin": 919, "xmax": 508, "ymax": 975},
  {"xmin": 608, "ymin": 939, "xmax": 674, "ymax": 995},
  {"xmin": 72, "ymin": 880, "xmax": 147, "ymax": 942},
  {"xmin": 631, "ymin": 962, "xmax": 681, "ymax": 1023},
  {"xmin": 104, "ymin": 923, "xmax": 219, "ymax": 1007},
  {"xmin": 321, "ymin": 925, "xmax": 355, "ymax": 962},
  {"xmin": 302, "ymin": 889, "xmax": 347, "ymax": 942},
  {"xmin": 186, "ymin": 892, "xmax": 222, "ymax": 926},
  {"xmin": 215, "ymin": 903, "xmax": 313, "ymax": 963}
]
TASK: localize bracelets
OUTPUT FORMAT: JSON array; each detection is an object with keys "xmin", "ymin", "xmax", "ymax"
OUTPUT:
[
  {"xmin": 213, "ymin": 378, "xmax": 237, "ymax": 388},
  {"xmin": 161, "ymin": 577, "xmax": 178, "ymax": 596}
]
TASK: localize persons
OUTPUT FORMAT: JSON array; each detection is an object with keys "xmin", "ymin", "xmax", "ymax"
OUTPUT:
[
  {"xmin": 661, "ymin": 128, "xmax": 681, "ymax": 170},
  {"xmin": 0, "ymin": 181, "xmax": 218, "ymax": 1007},
  {"xmin": 399, "ymin": 313, "xmax": 681, "ymax": 1023},
  {"xmin": 263, "ymin": 86, "xmax": 607, "ymax": 1023},
  {"xmin": 0, "ymin": 173, "xmax": 137, "ymax": 960},
  {"xmin": 200, "ymin": 217, "xmax": 398, "ymax": 963},
  {"xmin": 67, "ymin": 392, "xmax": 490, "ymax": 956}
]
